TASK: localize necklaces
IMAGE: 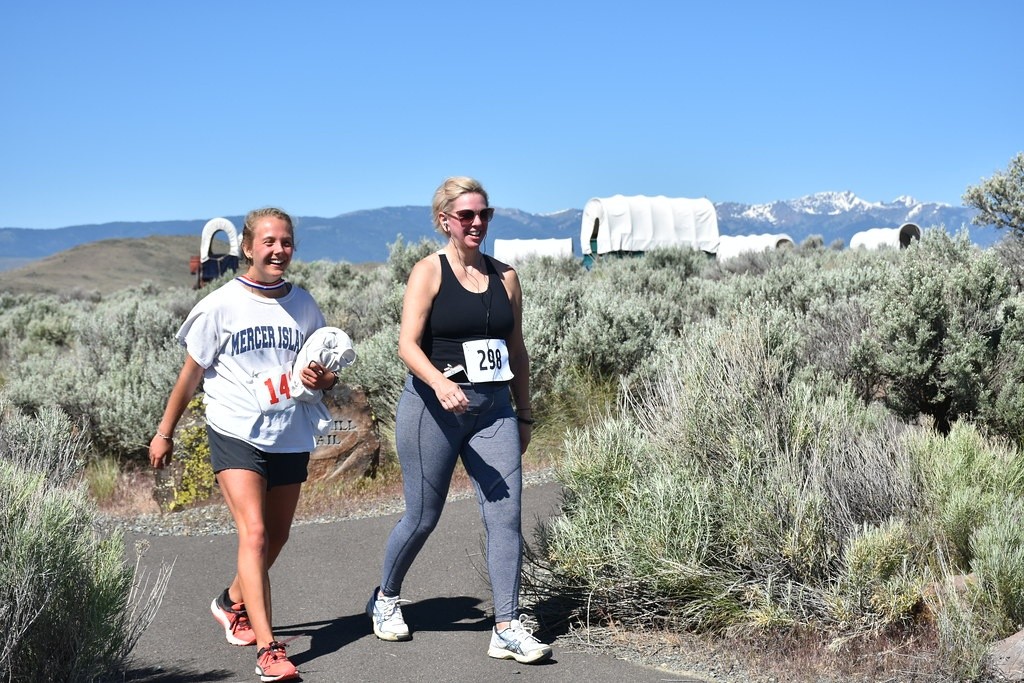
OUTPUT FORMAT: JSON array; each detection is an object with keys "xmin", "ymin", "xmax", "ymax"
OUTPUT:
[{"xmin": 236, "ymin": 276, "xmax": 285, "ymax": 290}]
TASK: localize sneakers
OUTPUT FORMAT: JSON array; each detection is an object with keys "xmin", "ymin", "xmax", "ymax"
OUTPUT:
[
  {"xmin": 255, "ymin": 640, "xmax": 299, "ymax": 682},
  {"xmin": 365, "ymin": 586, "xmax": 412, "ymax": 641},
  {"xmin": 210, "ymin": 587, "xmax": 257, "ymax": 645},
  {"xmin": 487, "ymin": 613, "xmax": 553, "ymax": 663}
]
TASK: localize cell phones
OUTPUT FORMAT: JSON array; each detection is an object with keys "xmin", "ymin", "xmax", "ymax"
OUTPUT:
[{"xmin": 443, "ymin": 365, "xmax": 470, "ymax": 384}]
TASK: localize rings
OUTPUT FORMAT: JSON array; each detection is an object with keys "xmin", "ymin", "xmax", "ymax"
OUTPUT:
[{"xmin": 307, "ymin": 375, "xmax": 309, "ymax": 380}]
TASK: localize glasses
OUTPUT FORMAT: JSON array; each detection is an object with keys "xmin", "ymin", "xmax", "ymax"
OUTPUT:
[{"xmin": 443, "ymin": 208, "xmax": 495, "ymax": 226}]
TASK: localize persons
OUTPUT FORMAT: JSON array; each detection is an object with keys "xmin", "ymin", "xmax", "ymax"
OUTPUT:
[
  {"xmin": 149, "ymin": 209, "xmax": 340, "ymax": 683},
  {"xmin": 366, "ymin": 176, "xmax": 553, "ymax": 663}
]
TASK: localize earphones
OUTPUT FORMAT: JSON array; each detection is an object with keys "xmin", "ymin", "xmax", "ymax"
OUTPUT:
[{"xmin": 444, "ymin": 221, "xmax": 448, "ymax": 228}]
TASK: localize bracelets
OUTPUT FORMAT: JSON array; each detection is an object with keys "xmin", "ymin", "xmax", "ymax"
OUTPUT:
[
  {"xmin": 157, "ymin": 430, "xmax": 174, "ymax": 439},
  {"xmin": 325, "ymin": 372, "xmax": 339, "ymax": 390}
]
